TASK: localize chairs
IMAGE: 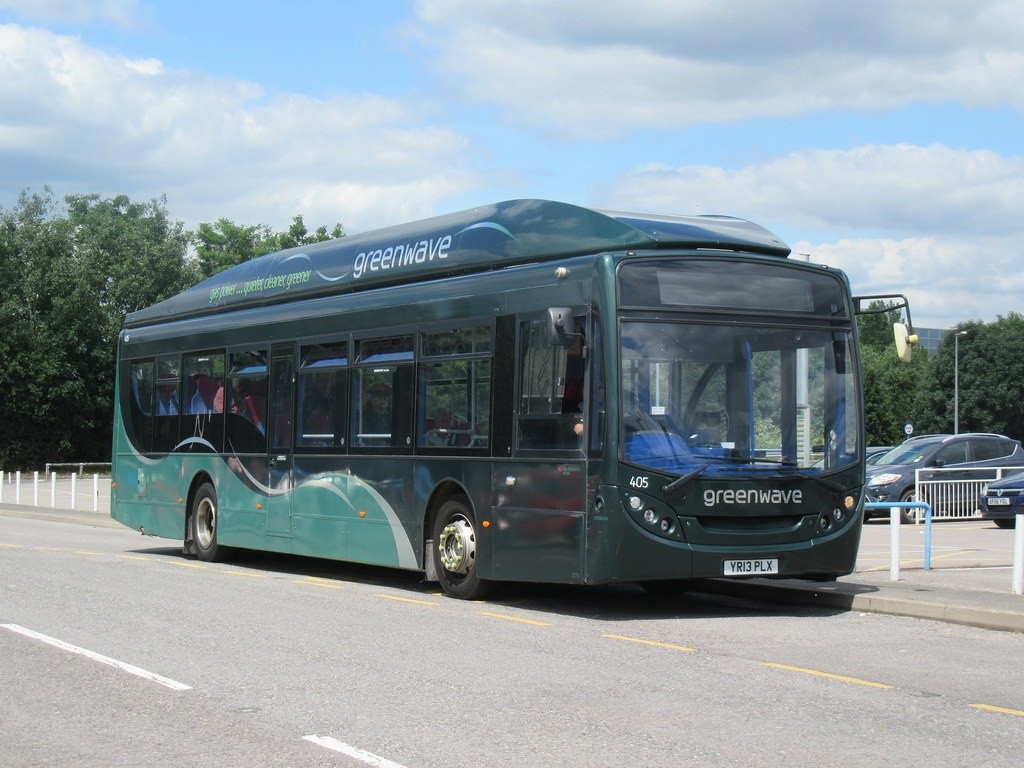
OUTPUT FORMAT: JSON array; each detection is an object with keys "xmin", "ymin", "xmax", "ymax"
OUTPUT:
[
  {"xmin": 132, "ymin": 373, "xmax": 489, "ymax": 449},
  {"xmin": 685, "ymin": 409, "xmax": 729, "ymax": 449}
]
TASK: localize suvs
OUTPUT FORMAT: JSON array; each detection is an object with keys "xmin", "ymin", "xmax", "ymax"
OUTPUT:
[{"xmin": 863, "ymin": 433, "xmax": 1024, "ymax": 523}]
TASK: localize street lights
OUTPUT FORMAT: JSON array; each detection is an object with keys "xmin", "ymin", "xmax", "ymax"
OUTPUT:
[{"xmin": 955, "ymin": 331, "xmax": 968, "ymax": 434}]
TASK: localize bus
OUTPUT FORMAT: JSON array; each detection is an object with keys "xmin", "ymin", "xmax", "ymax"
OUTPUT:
[{"xmin": 109, "ymin": 197, "xmax": 916, "ymax": 601}]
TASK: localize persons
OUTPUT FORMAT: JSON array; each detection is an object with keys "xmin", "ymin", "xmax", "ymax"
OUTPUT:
[
  {"xmin": 362, "ymin": 390, "xmax": 393, "ymax": 433},
  {"xmin": 422, "ymin": 408, "xmax": 456, "ymax": 446},
  {"xmin": 306, "ymin": 371, "xmax": 335, "ymax": 417},
  {"xmin": 573, "ymin": 381, "xmax": 606, "ymax": 437},
  {"xmin": 214, "ymin": 377, "xmax": 245, "ymax": 415}
]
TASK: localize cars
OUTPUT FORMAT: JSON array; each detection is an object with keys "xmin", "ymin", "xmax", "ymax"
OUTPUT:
[
  {"xmin": 811, "ymin": 446, "xmax": 895, "ymax": 469},
  {"xmin": 978, "ymin": 470, "xmax": 1024, "ymax": 529}
]
